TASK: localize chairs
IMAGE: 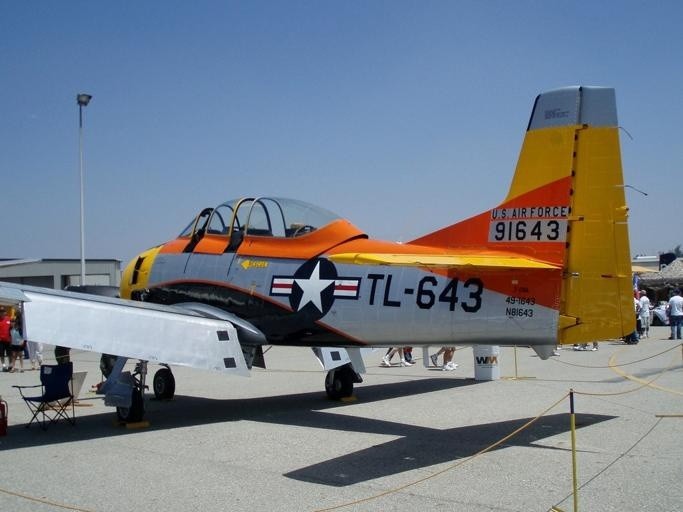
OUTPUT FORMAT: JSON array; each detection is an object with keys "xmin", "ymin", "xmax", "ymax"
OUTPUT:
[{"xmin": 10, "ymin": 361, "xmax": 75, "ymax": 443}]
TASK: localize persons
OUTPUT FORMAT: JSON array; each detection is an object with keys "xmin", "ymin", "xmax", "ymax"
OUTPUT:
[
  {"xmin": 39, "ymin": 346, "xmax": 71, "ymax": 399},
  {"xmin": 95, "ymin": 354, "xmax": 117, "ymax": 391},
  {"xmin": 0, "ymin": 305, "xmax": 42, "ymax": 373},
  {"xmin": 666, "ymin": 287, "xmax": 682, "ymax": 339},
  {"xmin": 381, "ymin": 347, "xmax": 458, "ymax": 371},
  {"xmin": 631, "ymin": 292, "xmax": 640, "ymax": 344},
  {"xmin": 573, "ymin": 340, "xmax": 598, "ymax": 352},
  {"xmin": 639, "ymin": 290, "xmax": 649, "ymax": 337}
]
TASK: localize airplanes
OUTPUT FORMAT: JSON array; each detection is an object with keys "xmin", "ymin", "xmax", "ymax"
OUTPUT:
[{"xmin": 0, "ymin": 85, "xmax": 637, "ymax": 426}]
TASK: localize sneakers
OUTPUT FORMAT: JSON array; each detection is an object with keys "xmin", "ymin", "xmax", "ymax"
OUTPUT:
[
  {"xmin": 380, "ymin": 354, "xmax": 459, "ymax": 372},
  {"xmin": 668, "ymin": 336, "xmax": 682, "ymax": 341},
  {"xmin": 551, "ymin": 344, "xmax": 603, "ymax": 358}
]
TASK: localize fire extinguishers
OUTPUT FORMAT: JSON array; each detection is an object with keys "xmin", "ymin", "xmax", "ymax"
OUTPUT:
[{"xmin": 0, "ymin": 396, "xmax": 8, "ymax": 436}]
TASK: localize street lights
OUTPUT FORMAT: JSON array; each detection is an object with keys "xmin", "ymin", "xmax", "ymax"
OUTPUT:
[{"xmin": 77, "ymin": 93, "xmax": 92, "ymax": 286}]
